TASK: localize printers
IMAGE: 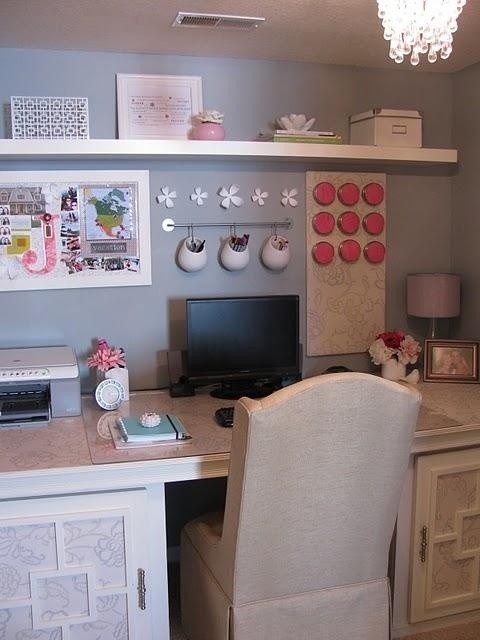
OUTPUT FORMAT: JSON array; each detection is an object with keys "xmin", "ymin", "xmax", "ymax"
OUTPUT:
[{"xmin": 1, "ymin": 346, "xmax": 84, "ymax": 427}]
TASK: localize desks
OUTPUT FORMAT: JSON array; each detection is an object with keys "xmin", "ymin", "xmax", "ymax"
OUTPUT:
[{"xmin": 1, "ymin": 367, "xmax": 480, "ymax": 640}]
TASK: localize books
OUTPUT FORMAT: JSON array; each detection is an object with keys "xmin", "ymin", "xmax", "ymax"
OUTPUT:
[
  {"xmin": 255, "ymin": 129, "xmax": 343, "ymax": 145},
  {"xmin": 108, "ymin": 412, "xmax": 193, "ymax": 450}
]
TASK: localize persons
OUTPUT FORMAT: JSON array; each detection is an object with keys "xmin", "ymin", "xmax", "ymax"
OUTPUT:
[
  {"xmin": 0, "ymin": 185, "xmax": 139, "ymax": 274},
  {"xmin": 432, "ymin": 347, "xmax": 473, "ymax": 375}
]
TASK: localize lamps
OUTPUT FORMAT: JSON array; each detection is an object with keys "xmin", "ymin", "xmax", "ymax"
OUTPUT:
[
  {"xmin": 376, "ymin": 0, "xmax": 467, "ymax": 68},
  {"xmin": 405, "ymin": 272, "xmax": 463, "ymax": 339}
]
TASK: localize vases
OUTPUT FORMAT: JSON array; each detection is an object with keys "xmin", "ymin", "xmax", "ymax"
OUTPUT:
[
  {"xmin": 96, "ymin": 368, "xmax": 130, "ymax": 401},
  {"xmin": 381, "ymin": 359, "xmax": 407, "ymax": 381}
]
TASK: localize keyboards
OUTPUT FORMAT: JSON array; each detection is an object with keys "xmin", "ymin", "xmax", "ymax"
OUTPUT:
[{"xmin": 214, "ymin": 403, "xmax": 234, "ymax": 427}]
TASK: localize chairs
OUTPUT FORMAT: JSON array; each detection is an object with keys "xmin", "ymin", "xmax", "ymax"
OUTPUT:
[{"xmin": 177, "ymin": 371, "xmax": 424, "ymax": 640}]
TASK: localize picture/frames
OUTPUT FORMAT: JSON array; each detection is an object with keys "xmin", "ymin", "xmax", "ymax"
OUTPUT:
[
  {"xmin": 423, "ymin": 337, "xmax": 480, "ymax": 384},
  {"xmin": 0, "ymin": 168, "xmax": 153, "ymax": 293},
  {"xmin": 114, "ymin": 71, "xmax": 205, "ymax": 140}
]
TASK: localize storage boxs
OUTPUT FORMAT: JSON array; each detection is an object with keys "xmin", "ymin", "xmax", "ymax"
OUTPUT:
[{"xmin": 349, "ymin": 107, "xmax": 423, "ymax": 148}]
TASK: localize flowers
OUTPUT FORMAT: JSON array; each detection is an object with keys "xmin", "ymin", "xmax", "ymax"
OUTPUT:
[
  {"xmin": 86, "ymin": 337, "xmax": 128, "ymax": 373},
  {"xmin": 369, "ymin": 330, "xmax": 422, "ymax": 368}
]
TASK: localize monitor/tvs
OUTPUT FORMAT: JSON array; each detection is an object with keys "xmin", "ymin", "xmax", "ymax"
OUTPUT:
[{"xmin": 183, "ymin": 294, "xmax": 301, "ymax": 400}]
